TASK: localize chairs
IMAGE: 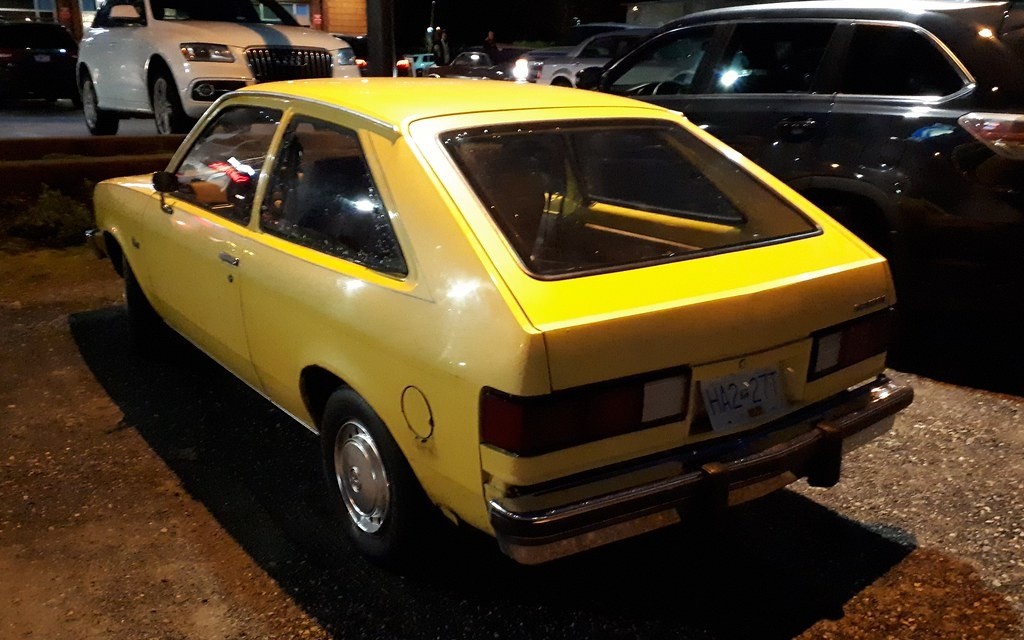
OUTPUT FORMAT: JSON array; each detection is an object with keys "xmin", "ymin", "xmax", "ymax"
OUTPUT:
[
  {"xmin": 492, "ymin": 141, "xmax": 545, "ymax": 254},
  {"xmin": 529, "ymin": 152, "xmax": 588, "ymax": 274},
  {"xmin": 283, "ymin": 156, "xmax": 372, "ymax": 227}
]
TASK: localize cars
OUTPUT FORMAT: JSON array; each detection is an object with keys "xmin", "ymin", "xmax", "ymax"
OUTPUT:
[
  {"xmin": 396, "ymin": 20, "xmax": 746, "ymax": 90},
  {"xmin": 577, "ymin": 0, "xmax": 1024, "ymax": 302},
  {"xmin": 86, "ymin": 74, "xmax": 917, "ymax": 571},
  {"xmin": 0, "ymin": 17, "xmax": 84, "ymax": 109},
  {"xmin": 74, "ymin": 0, "xmax": 363, "ymax": 138}
]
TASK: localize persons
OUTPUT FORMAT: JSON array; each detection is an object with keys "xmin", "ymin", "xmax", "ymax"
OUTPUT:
[
  {"xmin": 433, "ymin": 29, "xmax": 451, "ymax": 66},
  {"xmin": 483, "ymin": 31, "xmax": 499, "ymax": 65}
]
